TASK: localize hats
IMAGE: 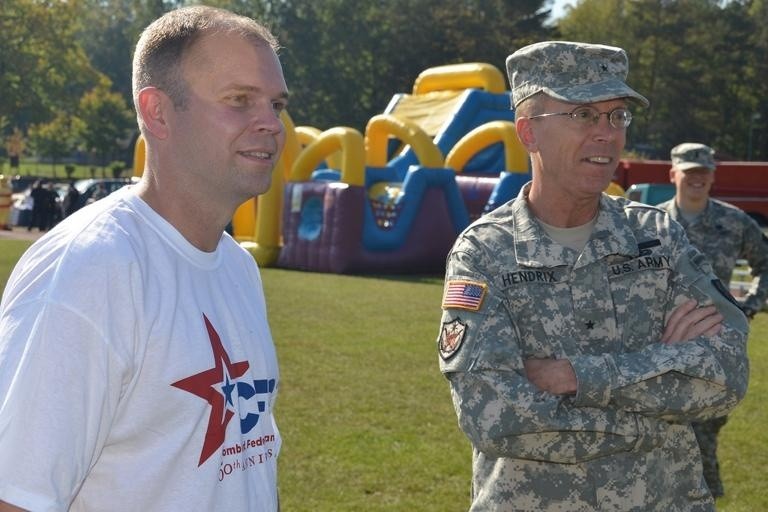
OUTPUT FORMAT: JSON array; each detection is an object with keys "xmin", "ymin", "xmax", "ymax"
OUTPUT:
[
  {"xmin": 505, "ymin": 40, "xmax": 650, "ymax": 112},
  {"xmin": 670, "ymin": 142, "xmax": 717, "ymax": 172}
]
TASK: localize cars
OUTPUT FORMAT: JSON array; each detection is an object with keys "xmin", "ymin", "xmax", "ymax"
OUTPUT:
[{"xmin": 12, "ymin": 177, "xmax": 132, "ymax": 220}]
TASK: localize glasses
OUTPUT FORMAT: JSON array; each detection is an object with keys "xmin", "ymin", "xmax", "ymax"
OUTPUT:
[{"xmin": 527, "ymin": 106, "xmax": 633, "ymax": 130}]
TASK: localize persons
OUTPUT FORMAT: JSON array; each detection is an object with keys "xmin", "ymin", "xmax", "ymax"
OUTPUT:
[
  {"xmin": 436, "ymin": 39, "xmax": 754, "ymax": 512},
  {"xmin": 1, "ymin": 6, "xmax": 294, "ymax": 509},
  {"xmin": 26, "ymin": 176, "xmax": 108, "ymax": 233},
  {"xmin": 652, "ymin": 142, "xmax": 768, "ymax": 496}
]
